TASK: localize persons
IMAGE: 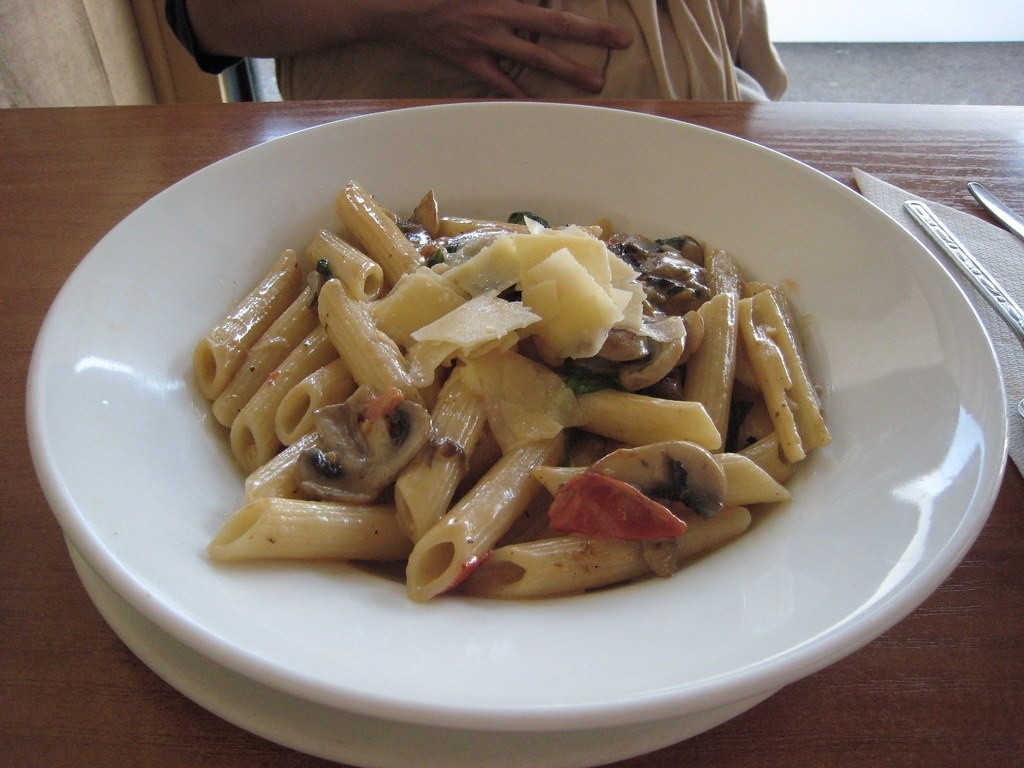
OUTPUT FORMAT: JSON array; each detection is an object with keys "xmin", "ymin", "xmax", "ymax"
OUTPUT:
[{"xmin": 156, "ymin": 0, "xmax": 789, "ymax": 102}]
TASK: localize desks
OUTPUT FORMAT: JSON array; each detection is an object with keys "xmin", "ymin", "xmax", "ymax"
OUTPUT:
[{"xmin": 0, "ymin": 99, "xmax": 1024, "ymax": 768}]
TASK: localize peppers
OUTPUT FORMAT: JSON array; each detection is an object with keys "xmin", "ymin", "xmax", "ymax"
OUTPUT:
[{"xmin": 550, "ymin": 472, "xmax": 686, "ymax": 538}]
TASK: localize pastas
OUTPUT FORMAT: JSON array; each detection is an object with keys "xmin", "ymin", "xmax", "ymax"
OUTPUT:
[{"xmin": 194, "ymin": 182, "xmax": 833, "ymax": 600}]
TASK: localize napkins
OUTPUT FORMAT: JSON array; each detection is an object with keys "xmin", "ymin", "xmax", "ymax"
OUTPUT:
[{"xmin": 850, "ymin": 166, "xmax": 1024, "ymax": 478}]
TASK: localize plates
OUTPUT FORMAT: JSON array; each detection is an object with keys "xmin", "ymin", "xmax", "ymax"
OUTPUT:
[
  {"xmin": 65, "ymin": 531, "xmax": 785, "ymax": 768},
  {"xmin": 25, "ymin": 102, "xmax": 1010, "ymax": 733}
]
